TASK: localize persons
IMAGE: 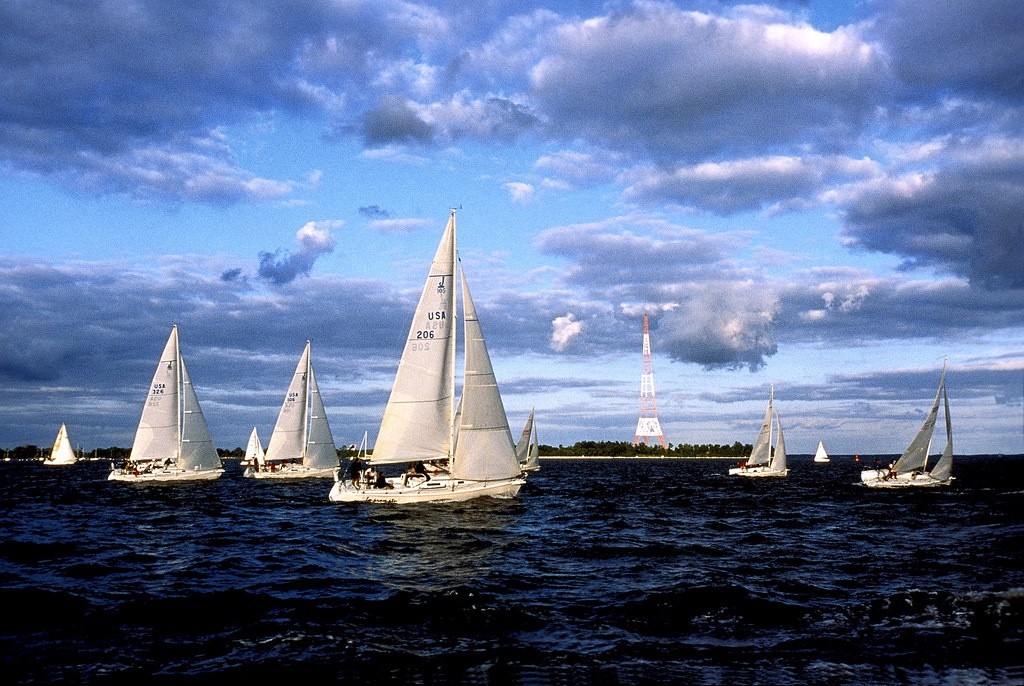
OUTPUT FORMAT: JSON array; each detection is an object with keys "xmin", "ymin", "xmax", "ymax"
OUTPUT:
[
  {"xmin": 338, "ymin": 452, "xmax": 393, "ymax": 490},
  {"xmin": 253, "ymin": 456, "xmax": 260, "ymax": 473},
  {"xmin": 872, "ymin": 459, "xmax": 888, "ymax": 482},
  {"xmin": 263, "ymin": 462, "xmax": 277, "ymax": 473},
  {"xmin": 119, "ymin": 460, "xmax": 152, "ymax": 475},
  {"xmin": 889, "ymin": 460, "xmax": 898, "ymax": 479},
  {"xmin": 163, "ymin": 457, "xmax": 173, "ymax": 473},
  {"xmin": 415, "ymin": 461, "xmax": 431, "ymax": 481}
]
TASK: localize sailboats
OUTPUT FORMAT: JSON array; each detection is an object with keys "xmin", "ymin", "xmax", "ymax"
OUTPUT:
[
  {"xmin": 239, "ymin": 425, "xmax": 267, "ymax": 468},
  {"xmin": 860, "ymin": 355, "xmax": 957, "ymax": 489},
  {"xmin": 106, "ymin": 323, "xmax": 225, "ymax": 484},
  {"xmin": 41, "ymin": 422, "xmax": 79, "ymax": 468},
  {"xmin": 243, "ymin": 339, "xmax": 342, "ymax": 480},
  {"xmin": 515, "ymin": 405, "xmax": 543, "ymax": 474},
  {"xmin": 814, "ymin": 439, "xmax": 832, "ymax": 463},
  {"xmin": 329, "ymin": 210, "xmax": 526, "ymax": 505},
  {"xmin": 728, "ymin": 382, "xmax": 790, "ymax": 478}
]
